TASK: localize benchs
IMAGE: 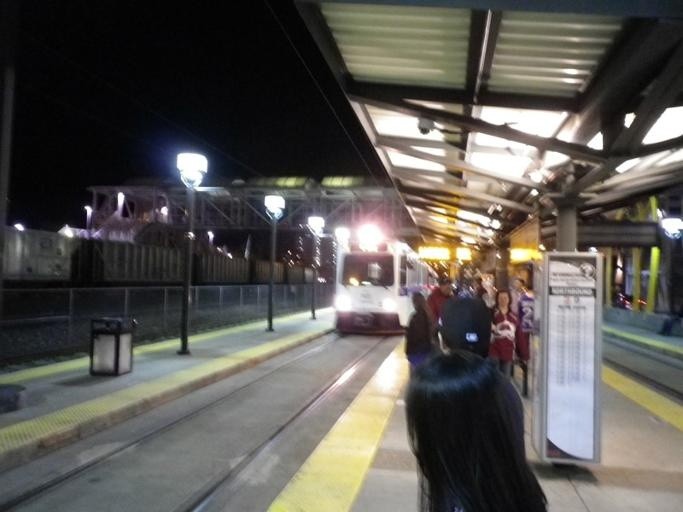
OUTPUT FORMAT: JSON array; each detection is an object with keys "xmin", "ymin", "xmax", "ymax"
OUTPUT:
[{"xmin": 601, "ymin": 302, "xmax": 682, "ymax": 396}]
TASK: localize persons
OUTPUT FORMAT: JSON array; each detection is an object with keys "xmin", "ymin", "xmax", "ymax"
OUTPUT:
[{"xmin": 404, "ymin": 260, "xmax": 548, "ymax": 512}]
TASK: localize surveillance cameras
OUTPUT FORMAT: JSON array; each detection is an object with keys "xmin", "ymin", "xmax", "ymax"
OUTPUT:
[{"xmin": 418, "ymin": 117, "xmax": 435, "ymax": 136}]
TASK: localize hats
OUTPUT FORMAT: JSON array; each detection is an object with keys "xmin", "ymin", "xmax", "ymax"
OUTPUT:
[{"xmin": 437, "ymin": 297, "xmax": 491, "ymax": 342}]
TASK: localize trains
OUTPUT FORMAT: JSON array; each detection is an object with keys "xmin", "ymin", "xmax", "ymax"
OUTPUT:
[{"xmin": 333, "ymin": 237, "xmax": 439, "ymax": 338}]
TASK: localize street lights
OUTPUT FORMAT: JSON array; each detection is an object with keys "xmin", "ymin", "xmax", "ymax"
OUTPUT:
[
  {"xmin": 660, "ymin": 215, "xmax": 682, "ymax": 337},
  {"xmin": 176, "ymin": 152, "xmax": 207, "ymax": 356},
  {"xmin": 334, "ymin": 226, "xmax": 349, "ymax": 245},
  {"xmin": 263, "ymin": 194, "xmax": 285, "ymax": 332},
  {"xmin": 307, "ymin": 216, "xmax": 324, "ymax": 320}
]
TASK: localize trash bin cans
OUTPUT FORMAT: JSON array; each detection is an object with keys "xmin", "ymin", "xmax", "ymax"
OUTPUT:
[{"xmin": 90, "ymin": 315, "xmax": 135, "ymax": 376}]
